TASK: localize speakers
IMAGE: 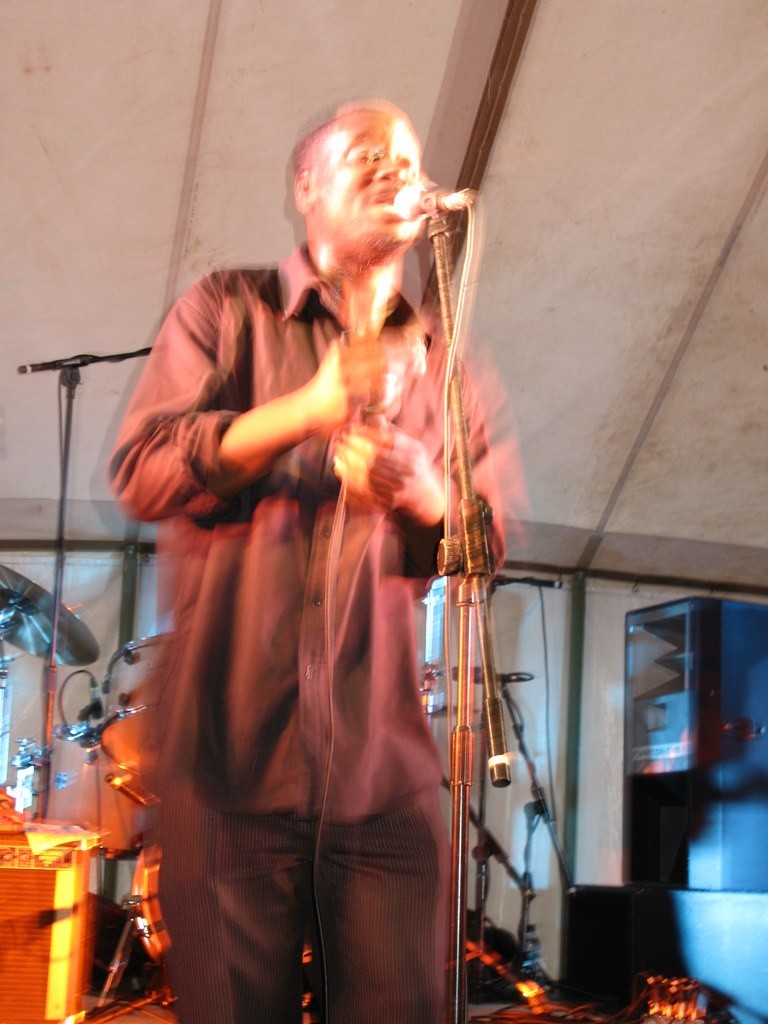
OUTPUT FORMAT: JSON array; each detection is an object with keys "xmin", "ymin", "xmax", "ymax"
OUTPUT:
[
  {"xmin": 624, "ymin": 597, "xmax": 768, "ymax": 894},
  {"xmin": 0, "ymin": 837, "xmax": 92, "ymax": 1024},
  {"xmin": 562, "ymin": 887, "xmax": 678, "ymax": 1012}
]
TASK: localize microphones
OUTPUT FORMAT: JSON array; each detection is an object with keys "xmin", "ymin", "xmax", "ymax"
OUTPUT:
[
  {"xmin": 393, "ymin": 185, "xmax": 471, "ymax": 223},
  {"xmin": 103, "ymin": 773, "xmax": 145, "ymax": 807},
  {"xmin": 452, "ymin": 667, "xmax": 528, "ymax": 684},
  {"xmin": 89, "ymin": 678, "xmax": 103, "ymax": 720}
]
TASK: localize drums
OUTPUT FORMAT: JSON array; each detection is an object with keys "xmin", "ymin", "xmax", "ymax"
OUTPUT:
[
  {"xmin": 100, "ymin": 631, "xmax": 177, "ymax": 775},
  {"xmin": 127, "ymin": 843, "xmax": 313, "ymax": 965}
]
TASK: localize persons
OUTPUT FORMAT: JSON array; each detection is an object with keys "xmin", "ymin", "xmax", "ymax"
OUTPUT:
[{"xmin": 106, "ymin": 93, "xmax": 516, "ymax": 1024}]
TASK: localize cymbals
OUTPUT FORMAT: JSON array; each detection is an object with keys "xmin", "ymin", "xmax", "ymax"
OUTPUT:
[{"xmin": 0, "ymin": 563, "xmax": 100, "ymax": 667}]
